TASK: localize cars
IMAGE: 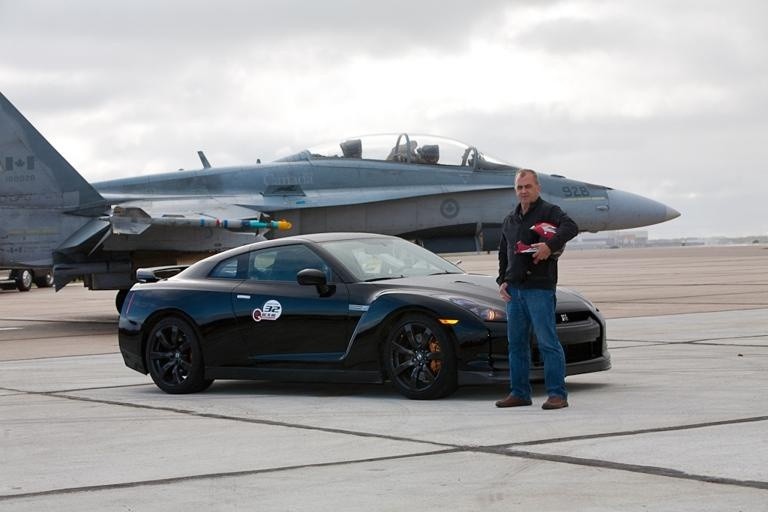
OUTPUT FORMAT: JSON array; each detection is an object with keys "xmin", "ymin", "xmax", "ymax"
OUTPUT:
[
  {"xmin": 0, "ymin": 267, "xmax": 54, "ymax": 291},
  {"xmin": 116, "ymin": 230, "xmax": 609, "ymax": 401}
]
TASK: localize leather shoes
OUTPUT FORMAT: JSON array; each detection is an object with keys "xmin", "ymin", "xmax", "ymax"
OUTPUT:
[
  {"xmin": 495, "ymin": 395, "xmax": 532, "ymax": 407},
  {"xmin": 542, "ymin": 396, "xmax": 568, "ymax": 409}
]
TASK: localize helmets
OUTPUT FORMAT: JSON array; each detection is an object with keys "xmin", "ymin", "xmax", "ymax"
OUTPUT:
[{"xmin": 514, "ymin": 223, "xmax": 566, "ymax": 261}]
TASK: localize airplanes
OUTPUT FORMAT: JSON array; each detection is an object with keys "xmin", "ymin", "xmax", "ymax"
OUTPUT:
[{"xmin": 0, "ymin": 89, "xmax": 689, "ymax": 313}]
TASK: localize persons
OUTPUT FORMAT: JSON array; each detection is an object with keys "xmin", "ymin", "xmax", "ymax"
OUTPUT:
[{"xmin": 495, "ymin": 169, "xmax": 579, "ymax": 409}]
